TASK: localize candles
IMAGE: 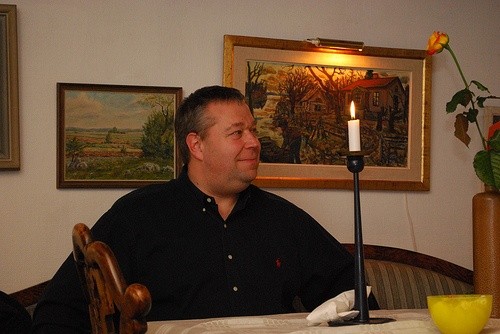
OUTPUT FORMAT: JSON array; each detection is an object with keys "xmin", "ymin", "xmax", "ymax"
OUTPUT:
[{"xmin": 347, "ymin": 100, "xmax": 361, "ymax": 152}]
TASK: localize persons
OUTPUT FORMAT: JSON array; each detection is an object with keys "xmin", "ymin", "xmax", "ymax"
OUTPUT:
[{"xmin": 33, "ymin": 86, "xmax": 381, "ymax": 334}]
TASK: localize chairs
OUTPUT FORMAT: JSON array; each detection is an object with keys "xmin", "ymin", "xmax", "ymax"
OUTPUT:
[{"xmin": 71, "ymin": 223, "xmax": 152, "ymax": 334}]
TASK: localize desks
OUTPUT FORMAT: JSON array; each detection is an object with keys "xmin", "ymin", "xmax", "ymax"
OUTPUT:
[{"xmin": 145, "ymin": 309, "xmax": 500, "ymax": 334}]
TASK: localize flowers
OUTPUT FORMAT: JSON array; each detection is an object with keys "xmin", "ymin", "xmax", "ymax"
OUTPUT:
[{"xmin": 427, "ymin": 31, "xmax": 500, "ymax": 190}]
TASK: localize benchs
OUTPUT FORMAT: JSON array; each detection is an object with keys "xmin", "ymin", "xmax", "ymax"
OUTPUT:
[{"xmin": 0, "ymin": 243, "xmax": 476, "ymax": 320}]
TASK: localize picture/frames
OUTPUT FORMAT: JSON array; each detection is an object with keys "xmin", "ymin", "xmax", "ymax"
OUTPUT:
[
  {"xmin": 482, "ymin": 106, "xmax": 500, "ymax": 145},
  {"xmin": 56, "ymin": 82, "xmax": 183, "ymax": 188},
  {"xmin": 222, "ymin": 34, "xmax": 433, "ymax": 192},
  {"xmin": 0, "ymin": 3, "xmax": 21, "ymax": 171}
]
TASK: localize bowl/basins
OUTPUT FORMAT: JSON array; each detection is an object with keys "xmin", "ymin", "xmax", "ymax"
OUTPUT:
[{"xmin": 427, "ymin": 294, "xmax": 493, "ymax": 334}]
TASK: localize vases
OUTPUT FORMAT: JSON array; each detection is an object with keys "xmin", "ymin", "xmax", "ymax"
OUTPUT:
[{"xmin": 471, "ymin": 185, "xmax": 500, "ymax": 319}]
